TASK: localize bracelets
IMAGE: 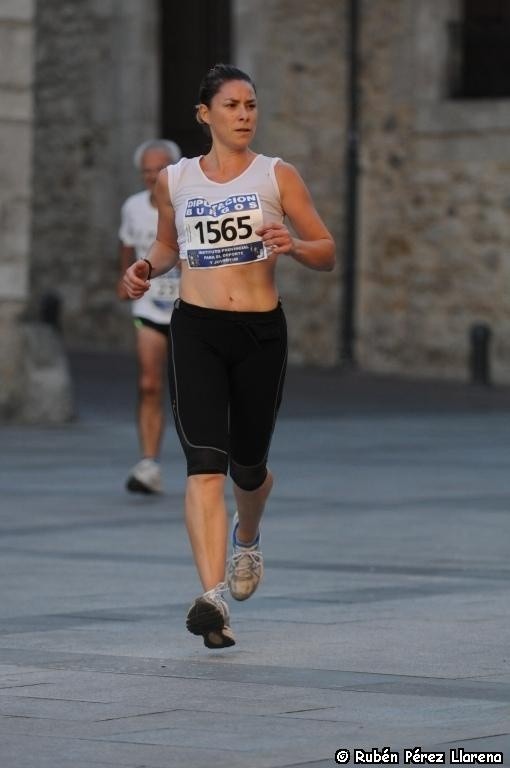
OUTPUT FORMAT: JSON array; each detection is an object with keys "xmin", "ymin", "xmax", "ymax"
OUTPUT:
[{"xmin": 141, "ymin": 259, "xmax": 153, "ymax": 280}]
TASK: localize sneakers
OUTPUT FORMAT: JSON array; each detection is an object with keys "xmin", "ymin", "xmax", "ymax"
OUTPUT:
[
  {"xmin": 185, "ymin": 594, "xmax": 236, "ymax": 650},
  {"xmin": 227, "ymin": 511, "xmax": 262, "ymax": 601},
  {"xmin": 126, "ymin": 459, "xmax": 163, "ymax": 494}
]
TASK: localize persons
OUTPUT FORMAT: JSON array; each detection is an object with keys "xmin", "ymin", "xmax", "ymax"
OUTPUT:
[
  {"xmin": 121, "ymin": 65, "xmax": 337, "ymax": 649},
  {"xmin": 119, "ymin": 138, "xmax": 184, "ymax": 496}
]
